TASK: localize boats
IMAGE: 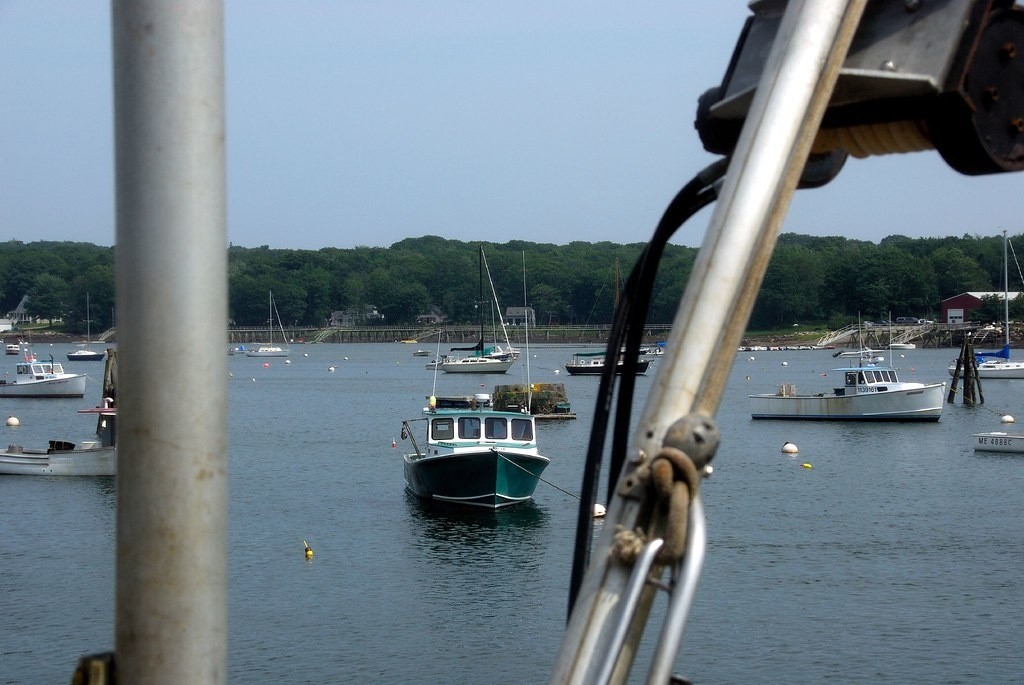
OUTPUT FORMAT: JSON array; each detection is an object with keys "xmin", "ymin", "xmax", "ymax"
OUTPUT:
[
  {"xmin": 971, "ymin": 432, "xmax": 1024, "ymax": 454},
  {"xmin": 245, "ymin": 290, "xmax": 291, "ymax": 357},
  {"xmin": 66, "ymin": 290, "xmax": 106, "ymax": 362},
  {"xmin": 402, "ymin": 310, "xmax": 550, "ymax": 511},
  {"xmin": 888, "ymin": 341, "xmax": 916, "ymax": 350},
  {"xmin": 5, "ymin": 344, "xmax": 20, "ymax": 355},
  {"xmin": 0, "ymin": 436, "xmax": 116, "ymax": 478},
  {"xmin": 0, "ymin": 327, "xmax": 88, "ymax": 398},
  {"xmin": 413, "ymin": 348, "xmax": 431, "ymax": 357},
  {"xmin": 748, "ymin": 308, "xmax": 947, "ymax": 422}
]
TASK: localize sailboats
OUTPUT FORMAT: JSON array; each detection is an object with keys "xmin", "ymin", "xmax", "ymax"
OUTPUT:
[
  {"xmin": 948, "ymin": 228, "xmax": 1024, "ymax": 380},
  {"xmin": 424, "ymin": 244, "xmax": 521, "ymax": 375},
  {"xmin": 565, "ymin": 253, "xmax": 655, "ymax": 375}
]
